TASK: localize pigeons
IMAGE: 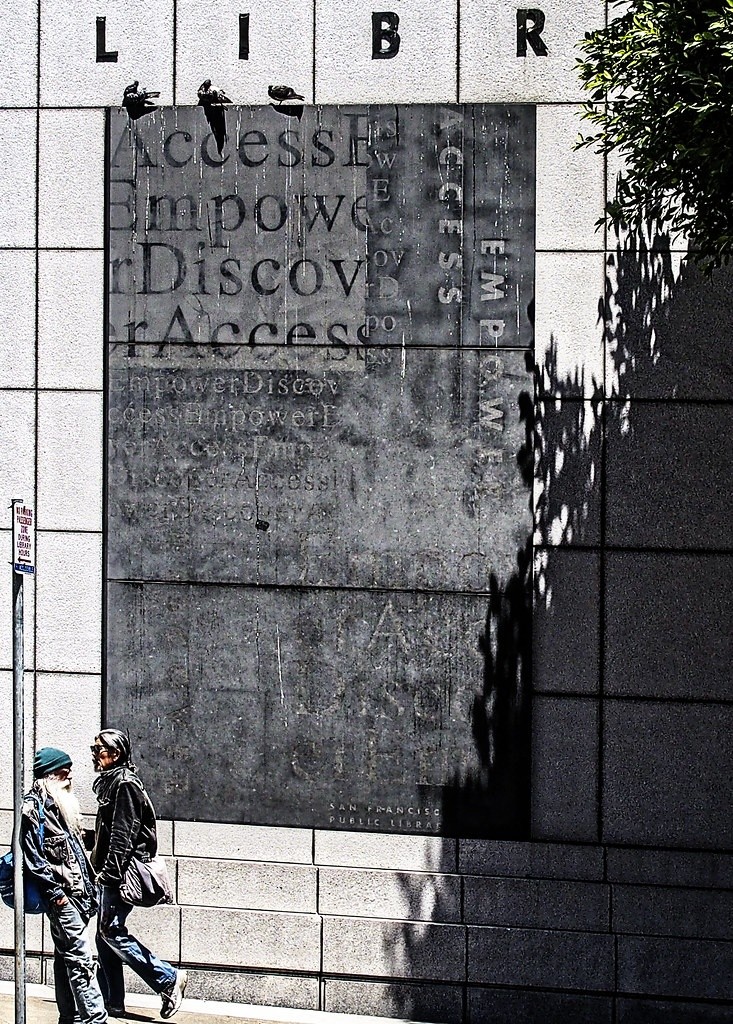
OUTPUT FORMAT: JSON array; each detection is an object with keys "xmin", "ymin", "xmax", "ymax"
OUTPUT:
[
  {"xmin": 268, "ymin": 85, "xmax": 305, "ymax": 105},
  {"xmin": 197, "ymin": 79, "xmax": 233, "ymax": 106},
  {"xmin": 123, "ymin": 81, "xmax": 160, "ymax": 107}
]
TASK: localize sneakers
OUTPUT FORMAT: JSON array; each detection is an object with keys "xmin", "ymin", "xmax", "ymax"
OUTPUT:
[{"xmin": 159, "ymin": 968, "xmax": 188, "ymax": 1019}]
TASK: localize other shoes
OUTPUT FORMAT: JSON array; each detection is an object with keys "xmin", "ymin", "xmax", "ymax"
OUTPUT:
[
  {"xmin": 57, "ymin": 1012, "xmax": 82, "ymax": 1022},
  {"xmin": 105, "ymin": 1005, "xmax": 126, "ymax": 1018}
]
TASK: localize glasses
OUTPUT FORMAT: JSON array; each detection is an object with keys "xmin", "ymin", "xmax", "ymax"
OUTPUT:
[{"xmin": 90, "ymin": 744, "xmax": 107, "ymax": 754}]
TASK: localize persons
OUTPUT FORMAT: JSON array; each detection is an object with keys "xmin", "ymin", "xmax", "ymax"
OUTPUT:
[
  {"xmin": 81, "ymin": 728, "xmax": 188, "ymax": 1019},
  {"xmin": 22, "ymin": 747, "xmax": 108, "ymax": 1024}
]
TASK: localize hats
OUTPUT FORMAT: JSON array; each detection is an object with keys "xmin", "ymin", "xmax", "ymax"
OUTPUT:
[{"xmin": 33, "ymin": 747, "xmax": 72, "ymax": 779}]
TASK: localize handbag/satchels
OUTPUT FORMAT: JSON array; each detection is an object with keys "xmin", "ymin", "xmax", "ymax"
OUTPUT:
[
  {"xmin": 118, "ymin": 855, "xmax": 175, "ymax": 908},
  {"xmin": 0, "ymin": 852, "xmax": 51, "ymax": 914}
]
